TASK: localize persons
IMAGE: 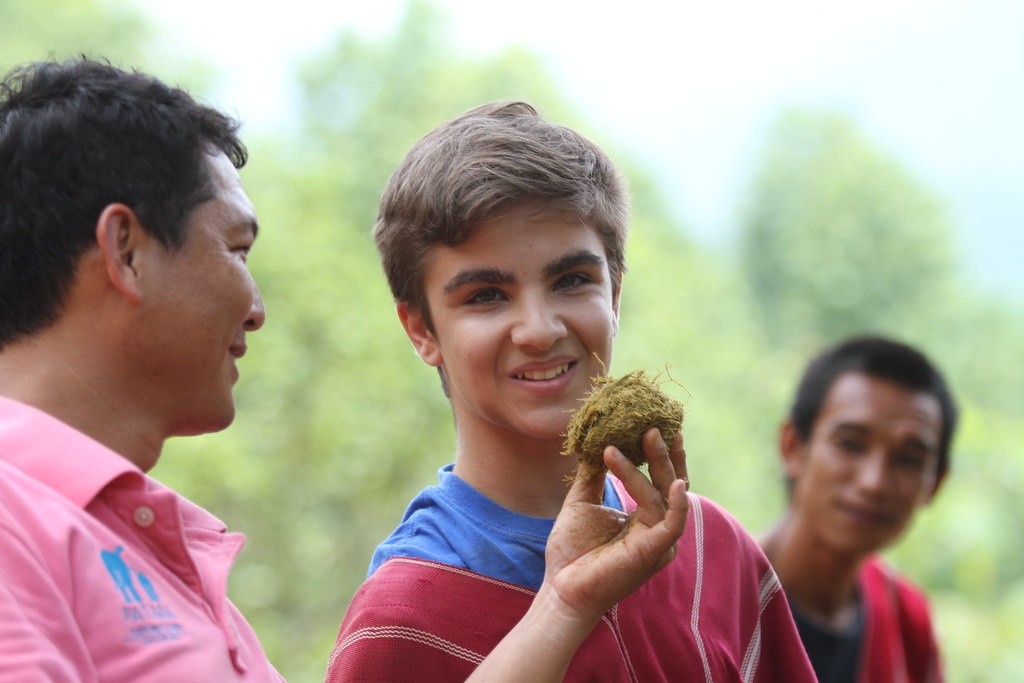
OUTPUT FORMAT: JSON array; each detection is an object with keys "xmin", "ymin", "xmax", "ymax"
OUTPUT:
[
  {"xmin": 325, "ymin": 99, "xmax": 818, "ymax": 683},
  {"xmin": 0, "ymin": 63, "xmax": 689, "ymax": 683},
  {"xmin": 750, "ymin": 337, "xmax": 957, "ymax": 683}
]
